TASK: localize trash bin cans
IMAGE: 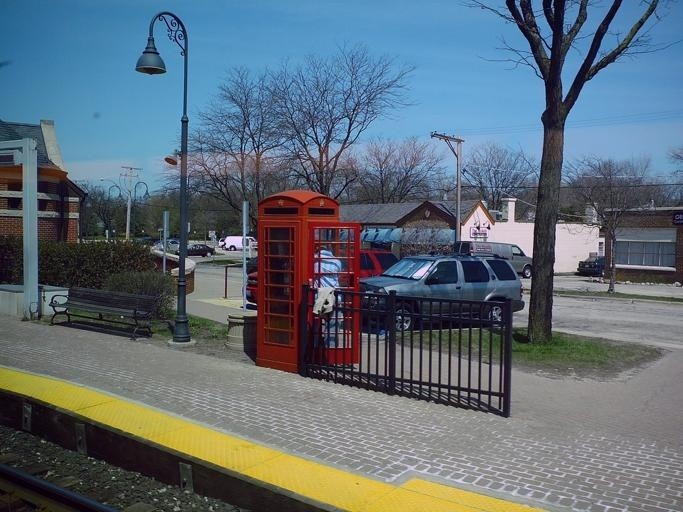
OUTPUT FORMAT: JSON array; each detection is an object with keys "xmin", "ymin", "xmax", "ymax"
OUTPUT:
[{"xmin": 38, "ymin": 286, "xmax": 45, "ymax": 316}]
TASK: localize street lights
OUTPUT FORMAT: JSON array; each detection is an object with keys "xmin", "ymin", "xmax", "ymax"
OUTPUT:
[
  {"xmin": 108, "ymin": 181, "xmax": 151, "ymax": 239},
  {"xmin": 135, "ymin": 11, "xmax": 189, "ymax": 343}
]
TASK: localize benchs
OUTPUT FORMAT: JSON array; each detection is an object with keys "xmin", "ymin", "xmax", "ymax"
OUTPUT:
[{"xmin": 49, "ymin": 286, "xmax": 160, "ymax": 341}]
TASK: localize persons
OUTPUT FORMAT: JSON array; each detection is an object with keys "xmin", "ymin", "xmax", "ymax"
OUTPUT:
[{"xmin": 314, "ymin": 238, "xmax": 344, "ymax": 348}]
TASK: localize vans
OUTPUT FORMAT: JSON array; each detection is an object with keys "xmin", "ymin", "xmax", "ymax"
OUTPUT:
[
  {"xmin": 219, "ymin": 235, "xmax": 257, "ymax": 252},
  {"xmin": 245, "ymin": 241, "xmax": 534, "ymax": 331}
]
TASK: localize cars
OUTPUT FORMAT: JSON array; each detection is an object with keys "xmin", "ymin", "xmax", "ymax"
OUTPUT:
[
  {"xmin": 577, "ymin": 256, "xmax": 605, "ymax": 276},
  {"xmin": 152, "ymin": 240, "xmax": 216, "ymax": 257}
]
